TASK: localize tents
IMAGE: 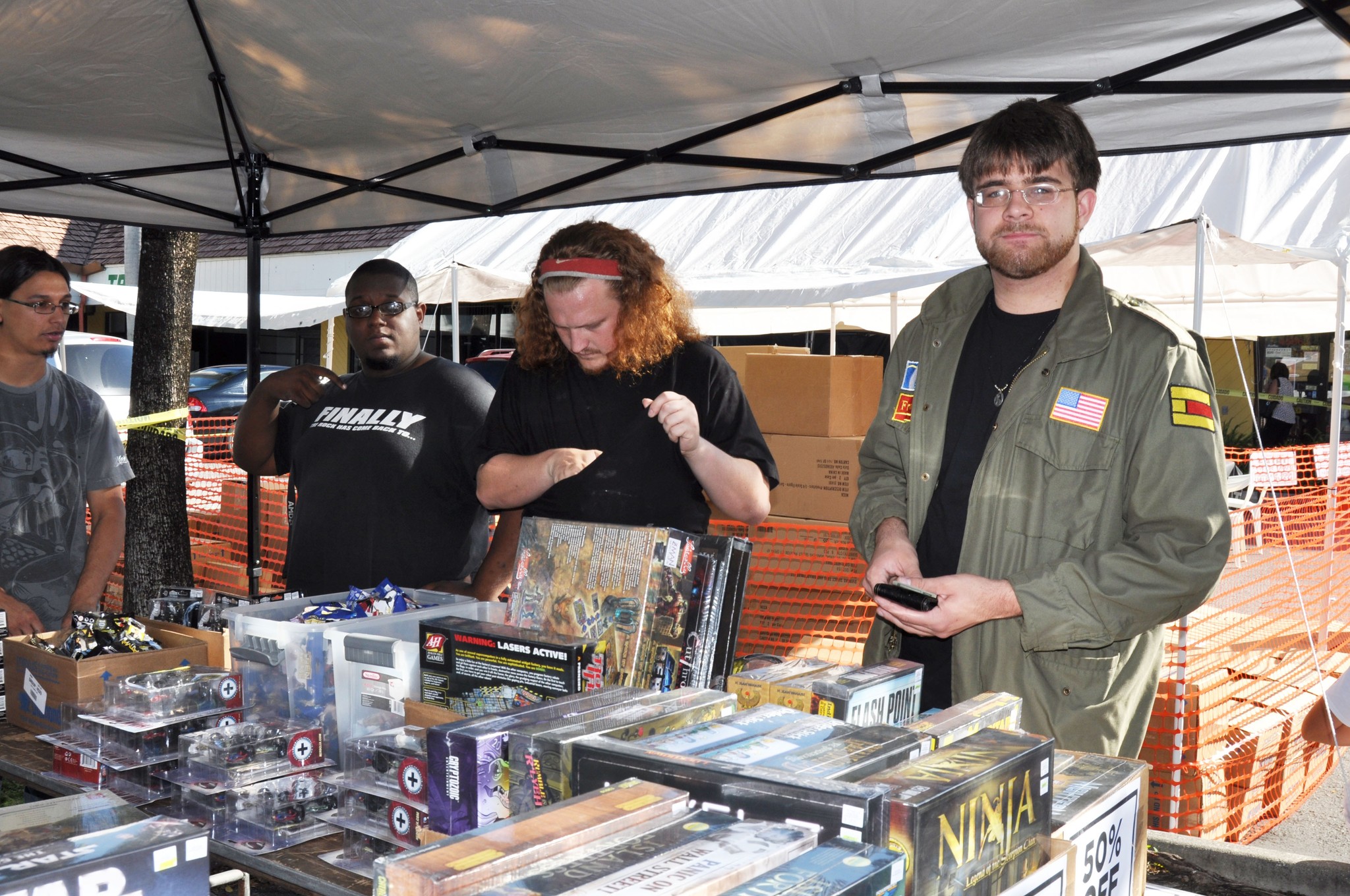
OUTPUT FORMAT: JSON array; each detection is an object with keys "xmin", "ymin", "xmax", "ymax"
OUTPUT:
[
  {"xmin": 0, "ymin": 0, "xmax": 1350, "ymax": 610},
  {"xmin": 322, "ymin": 128, "xmax": 1350, "ymax": 831}
]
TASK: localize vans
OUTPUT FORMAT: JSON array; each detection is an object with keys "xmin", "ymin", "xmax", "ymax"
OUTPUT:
[{"xmin": 45, "ymin": 329, "xmax": 136, "ymax": 443}]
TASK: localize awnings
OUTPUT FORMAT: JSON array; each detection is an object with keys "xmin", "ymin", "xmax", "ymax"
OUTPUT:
[{"xmin": 53, "ymin": 246, "xmax": 399, "ymax": 383}]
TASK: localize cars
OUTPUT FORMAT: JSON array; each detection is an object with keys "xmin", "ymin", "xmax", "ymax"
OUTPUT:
[{"xmin": 188, "ymin": 363, "xmax": 293, "ymax": 469}]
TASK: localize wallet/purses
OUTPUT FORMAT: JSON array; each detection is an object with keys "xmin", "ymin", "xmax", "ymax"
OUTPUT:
[{"xmin": 874, "ymin": 583, "xmax": 938, "ymax": 611}]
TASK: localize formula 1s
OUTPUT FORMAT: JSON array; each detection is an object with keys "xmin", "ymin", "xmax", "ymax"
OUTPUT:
[{"xmin": 79, "ymin": 664, "xmax": 428, "ymax": 854}]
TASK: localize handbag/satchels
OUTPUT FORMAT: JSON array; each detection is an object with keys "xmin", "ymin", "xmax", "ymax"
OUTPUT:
[{"xmin": 1259, "ymin": 378, "xmax": 1281, "ymax": 419}]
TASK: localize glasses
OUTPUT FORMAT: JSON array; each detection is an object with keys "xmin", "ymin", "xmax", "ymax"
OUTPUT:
[
  {"xmin": 0, "ymin": 296, "xmax": 79, "ymax": 315},
  {"xmin": 345, "ymin": 301, "xmax": 416, "ymax": 318},
  {"xmin": 971, "ymin": 184, "xmax": 1078, "ymax": 207}
]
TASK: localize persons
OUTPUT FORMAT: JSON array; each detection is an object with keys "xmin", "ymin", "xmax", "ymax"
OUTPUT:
[
  {"xmin": 231, "ymin": 259, "xmax": 524, "ymax": 611},
  {"xmin": 849, "ymin": 97, "xmax": 1235, "ymax": 759},
  {"xmin": 0, "ymin": 246, "xmax": 139, "ymax": 643},
  {"xmin": 473, "ymin": 219, "xmax": 780, "ymax": 539},
  {"xmin": 1257, "ymin": 362, "xmax": 1297, "ymax": 449}
]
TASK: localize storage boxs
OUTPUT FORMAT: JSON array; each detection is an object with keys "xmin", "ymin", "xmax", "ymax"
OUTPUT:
[
  {"xmin": 745, "ymin": 351, "xmax": 884, "ymax": 437},
  {"xmin": 712, "ymin": 343, "xmax": 810, "ymax": 392},
  {"xmin": 0, "ymin": 435, "xmax": 1350, "ymax": 896}
]
